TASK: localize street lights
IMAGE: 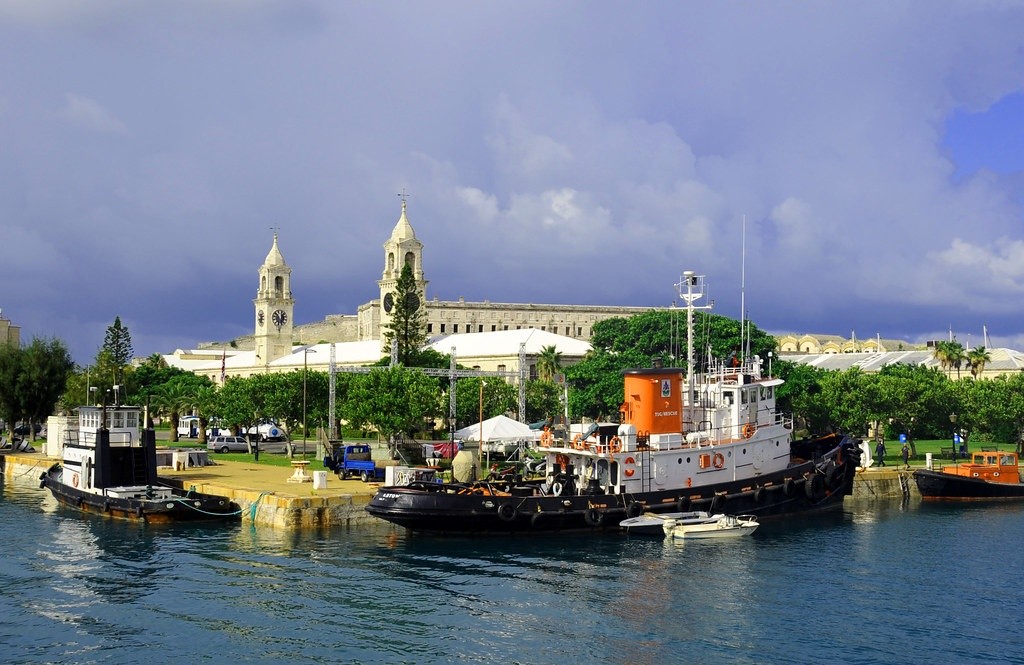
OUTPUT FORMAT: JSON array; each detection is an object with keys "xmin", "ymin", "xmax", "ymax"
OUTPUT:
[
  {"xmin": 949, "ymin": 412, "xmax": 957, "ymax": 461},
  {"xmin": 303, "ymin": 348, "xmax": 316, "ymax": 460},
  {"xmin": 447, "ymin": 414, "xmax": 456, "ymax": 460}
]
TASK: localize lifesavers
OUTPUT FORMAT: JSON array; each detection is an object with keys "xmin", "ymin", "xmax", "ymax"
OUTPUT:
[
  {"xmin": 76, "ymin": 496, "xmax": 82, "ymax": 506},
  {"xmin": 783, "ymin": 480, "xmax": 796, "ymax": 497},
  {"xmin": 541, "ymin": 432, "xmax": 551, "ymax": 447},
  {"xmin": 39, "ymin": 472, "xmax": 46, "ymax": 480},
  {"xmin": 627, "ymin": 502, "xmax": 644, "ymax": 518},
  {"xmin": 677, "ymin": 496, "xmax": 693, "ymax": 511},
  {"xmin": 714, "ymin": 495, "xmax": 728, "ymax": 511},
  {"xmin": 584, "ymin": 508, "xmax": 603, "ymax": 527},
  {"xmin": 136, "ymin": 507, "xmax": 141, "ymax": 517},
  {"xmin": 497, "ymin": 502, "xmax": 518, "ymax": 523},
  {"xmin": 73, "ymin": 473, "xmax": 78, "ymax": 487},
  {"xmin": 713, "ymin": 453, "xmax": 724, "ymax": 468},
  {"xmin": 40, "ymin": 481, "xmax": 45, "ymax": 488},
  {"xmin": 624, "ymin": 457, "xmax": 635, "ymax": 476},
  {"xmin": 610, "ymin": 437, "xmax": 622, "ymax": 452},
  {"xmin": 824, "ymin": 465, "xmax": 839, "ymax": 490},
  {"xmin": 573, "ymin": 433, "xmax": 585, "ymax": 450},
  {"xmin": 531, "ymin": 512, "xmax": 551, "ymax": 530},
  {"xmin": 103, "ymin": 502, "xmax": 110, "ymax": 512},
  {"xmin": 754, "ymin": 488, "xmax": 768, "ymax": 505},
  {"xmin": 744, "ymin": 423, "xmax": 753, "ymax": 438},
  {"xmin": 804, "ymin": 472, "xmax": 824, "ymax": 499}
]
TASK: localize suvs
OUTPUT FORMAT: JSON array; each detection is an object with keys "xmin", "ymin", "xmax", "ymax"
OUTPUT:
[
  {"xmin": 207, "ymin": 435, "xmax": 260, "ymax": 454},
  {"xmin": 14, "ymin": 424, "xmax": 41, "ymax": 435}
]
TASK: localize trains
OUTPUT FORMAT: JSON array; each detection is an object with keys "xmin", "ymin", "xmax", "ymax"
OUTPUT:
[{"xmin": 178, "ymin": 416, "xmax": 282, "ymax": 442}]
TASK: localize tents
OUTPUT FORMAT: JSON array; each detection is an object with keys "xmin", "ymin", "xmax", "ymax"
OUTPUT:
[{"xmin": 448, "ymin": 415, "xmax": 562, "ymax": 468}]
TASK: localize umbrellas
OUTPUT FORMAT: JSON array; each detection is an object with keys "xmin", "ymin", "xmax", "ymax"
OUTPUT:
[{"xmin": 452, "ymin": 450, "xmax": 481, "ymax": 482}]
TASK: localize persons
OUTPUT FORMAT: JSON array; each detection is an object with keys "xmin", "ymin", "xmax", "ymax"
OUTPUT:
[
  {"xmin": 899, "ymin": 443, "xmax": 911, "ymax": 469},
  {"xmin": 875, "ymin": 438, "xmax": 887, "ymax": 467},
  {"xmin": 960, "ymin": 444, "xmax": 965, "ymax": 457}
]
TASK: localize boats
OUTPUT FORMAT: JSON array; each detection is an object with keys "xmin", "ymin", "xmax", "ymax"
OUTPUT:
[
  {"xmin": 673, "ymin": 515, "xmax": 760, "ymax": 538},
  {"xmin": 364, "ymin": 215, "xmax": 860, "ymax": 536},
  {"xmin": 619, "ymin": 511, "xmax": 711, "ymax": 534},
  {"xmin": 911, "ymin": 450, "xmax": 1024, "ymax": 498},
  {"xmin": 44, "ymin": 365, "xmax": 232, "ymax": 523}
]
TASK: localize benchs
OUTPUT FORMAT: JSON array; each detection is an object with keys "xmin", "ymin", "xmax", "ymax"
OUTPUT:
[
  {"xmin": 981, "ymin": 446, "xmax": 997, "ymax": 452},
  {"xmin": 940, "ymin": 446, "xmax": 956, "ymax": 456}
]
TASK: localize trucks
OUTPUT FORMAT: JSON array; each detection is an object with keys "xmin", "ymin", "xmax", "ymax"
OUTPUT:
[{"xmin": 324, "ymin": 444, "xmax": 385, "ymax": 482}]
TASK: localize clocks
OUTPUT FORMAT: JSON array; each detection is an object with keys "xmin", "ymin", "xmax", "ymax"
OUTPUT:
[
  {"xmin": 257, "ymin": 311, "xmax": 265, "ymax": 326},
  {"xmin": 272, "ymin": 310, "xmax": 287, "ymax": 326},
  {"xmin": 384, "ymin": 294, "xmax": 392, "ymax": 312},
  {"xmin": 404, "ymin": 293, "xmax": 420, "ymax": 312}
]
